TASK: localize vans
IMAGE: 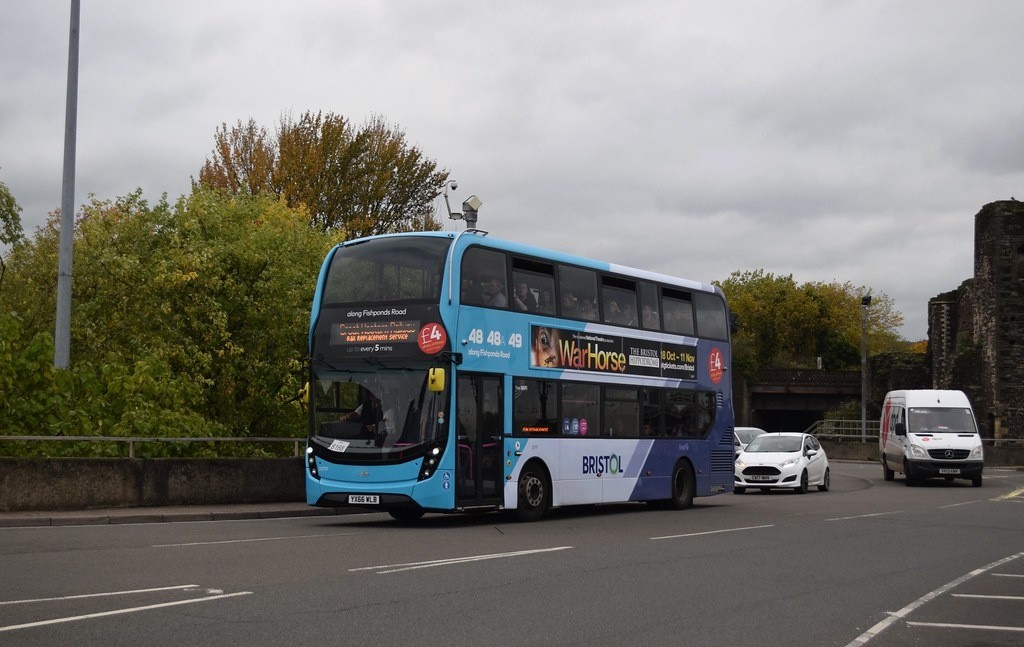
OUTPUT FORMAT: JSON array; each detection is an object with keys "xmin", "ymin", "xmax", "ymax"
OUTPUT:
[{"xmin": 878, "ymin": 389, "xmax": 984, "ymax": 489}]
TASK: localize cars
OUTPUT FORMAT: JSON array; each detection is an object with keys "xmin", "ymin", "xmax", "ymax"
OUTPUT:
[
  {"xmin": 733, "ymin": 432, "xmax": 830, "ymax": 497},
  {"xmin": 735, "ymin": 426, "xmax": 769, "ymax": 464}
]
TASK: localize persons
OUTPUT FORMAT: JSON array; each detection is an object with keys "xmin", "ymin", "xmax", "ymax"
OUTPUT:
[
  {"xmin": 340, "ymin": 384, "xmax": 397, "ymax": 435},
  {"xmin": 698, "ymin": 314, "xmax": 728, "ymax": 339},
  {"xmin": 645, "ymin": 393, "xmax": 711, "ymax": 437},
  {"xmin": 641, "ymin": 302, "xmax": 660, "ymax": 329},
  {"xmin": 603, "ymin": 298, "xmax": 637, "ymax": 326},
  {"xmin": 514, "ymin": 281, "xmax": 554, "ymax": 315},
  {"xmin": 912, "ymin": 415, "xmax": 929, "ymax": 431},
  {"xmin": 663, "ymin": 307, "xmax": 693, "ymax": 335},
  {"xmin": 562, "ymin": 293, "xmax": 598, "ymax": 321},
  {"xmin": 488, "ymin": 277, "xmax": 508, "ymax": 307}
]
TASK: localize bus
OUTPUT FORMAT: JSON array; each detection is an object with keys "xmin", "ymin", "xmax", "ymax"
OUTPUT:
[{"xmin": 304, "ymin": 229, "xmax": 735, "ymax": 522}]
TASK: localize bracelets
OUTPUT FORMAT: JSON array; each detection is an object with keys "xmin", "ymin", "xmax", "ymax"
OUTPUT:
[{"xmin": 515, "ymin": 296, "xmax": 518, "ymax": 299}]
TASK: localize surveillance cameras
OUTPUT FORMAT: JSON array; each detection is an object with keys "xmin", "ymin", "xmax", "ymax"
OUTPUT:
[{"xmin": 451, "ymin": 182, "xmax": 458, "ymax": 190}]
